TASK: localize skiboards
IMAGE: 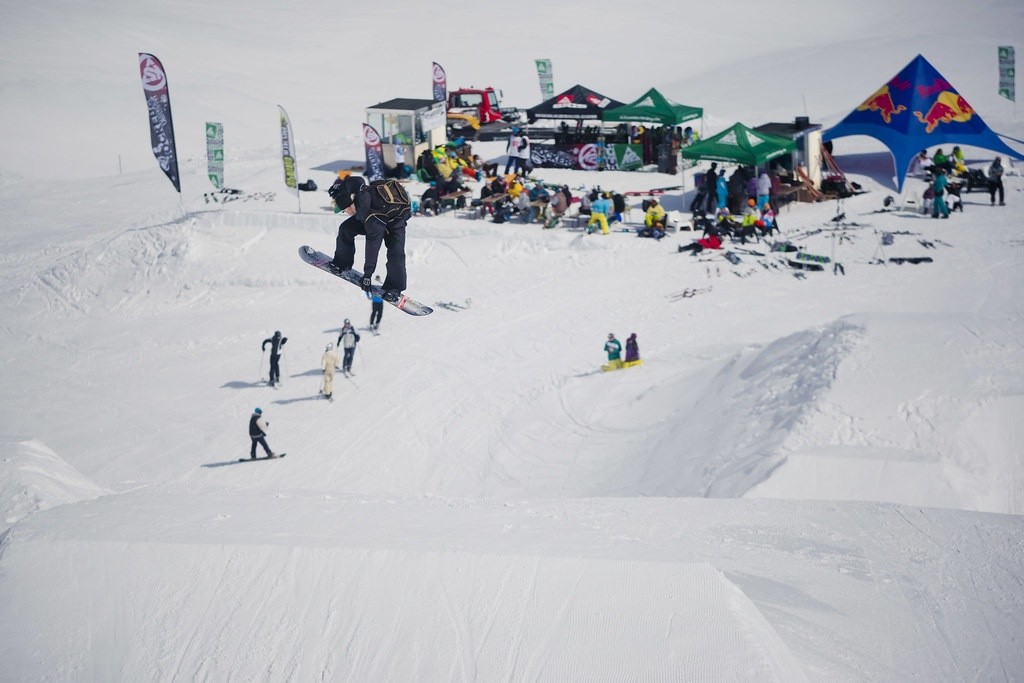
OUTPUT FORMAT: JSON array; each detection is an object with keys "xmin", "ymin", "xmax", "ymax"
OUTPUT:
[
  {"xmin": 787, "ymin": 219, "xmax": 954, "ymax": 249},
  {"xmin": 261, "ymin": 377, "xmax": 283, "ymax": 390},
  {"xmin": 663, "ymin": 286, "xmax": 714, "ymax": 303},
  {"xmin": 204, "ymin": 191, "xmax": 275, "ymax": 203},
  {"xmin": 320, "ymin": 389, "xmax": 334, "ymax": 403},
  {"xmin": 732, "ymin": 247, "xmax": 765, "ymax": 256},
  {"xmin": 790, "ymin": 270, "xmax": 807, "ymax": 280},
  {"xmin": 338, "ymin": 366, "xmax": 355, "ymax": 378},
  {"xmin": 699, "ymin": 258, "xmax": 787, "ymax": 281},
  {"xmin": 368, "ymin": 322, "xmax": 380, "ymax": 336}
]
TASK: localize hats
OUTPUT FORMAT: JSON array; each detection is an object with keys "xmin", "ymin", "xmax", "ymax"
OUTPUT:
[{"xmin": 335, "ymin": 191, "xmax": 354, "ymax": 210}]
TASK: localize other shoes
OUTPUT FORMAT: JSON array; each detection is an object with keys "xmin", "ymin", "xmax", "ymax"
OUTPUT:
[
  {"xmin": 329, "ymin": 261, "xmax": 352, "ymax": 274},
  {"xmin": 383, "ymin": 288, "xmax": 401, "ymax": 302}
]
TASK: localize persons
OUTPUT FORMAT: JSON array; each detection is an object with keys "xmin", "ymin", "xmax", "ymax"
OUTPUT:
[
  {"xmin": 624, "ymin": 333, "xmax": 640, "ymax": 367},
  {"xmin": 552, "ymin": 120, "xmax": 602, "ymax": 146},
  {"xmin": 261, "ymin": 330, "xmax": 287, "ymax": 385},
  {"xmin": 326, "ymin": 171, "xmax": 414, "ymax": 302},
  {"xmin": 637, "ymin": 197, "xmax": 666, "ymax": 239},
  {"xmin": 504, "ymin": 126, "xmax": 531, "ymax": 179},
  {"xmin": 679, "ymin": 234, "xmax": 723, "ymax": 255},
  {"xmin": 249, "ymin": 406, "xmax": 276, "ymax": 460},
  {"xmin": 340, "ymin": 320, "xmax": 359, "ymax": 373},
  {"xmin": 916, "ymin": 143, "xmax": 968, "ymax": 218},
  {"xmin": 618, "ymin": 123, "xmax": 703, "ymax": 165},
  {"xmin": 989, "ymin": 156, "xmax": 1007, "ymax": 205},
  {"xmin": 366, "ymin": 276, "xmax": 384, "ymax": 331},
  {"xmin": 321, "ymin": 343, "xmax": 341, "ymax": 397},
  {"xmin": 691, "ymin": 157, "xmax": 780, "ymax": 235},
  {"xmin": 452, "ymin": 153, "xmax": 626, "ymax": 235},
  {"xmin": 600, "ymin": 334, "xmax": 621, "ymax": 370}
]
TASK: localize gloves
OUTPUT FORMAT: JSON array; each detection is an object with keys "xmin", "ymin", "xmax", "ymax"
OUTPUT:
[
  {"xmin": 358, "ymin": 276, "xmax": 372, "ymax": 293},
  {"xmin": 327, "ymin": 184, "xmax": 344, "ymax": 198}
]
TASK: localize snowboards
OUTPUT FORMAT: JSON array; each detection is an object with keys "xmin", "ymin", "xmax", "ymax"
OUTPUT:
[
  {"xmin": 600, "ymin": 364, "xmax": 609, "ymax": 371},
  {"xmin": 889, "ymin": 257, "xmax": 933, "ymax": 265},
  {"xmin": 298, "ymin": 245, "xmax": 434, "ymax": 317},
  {"xmin": 655, "ymin": 186, "xmax": 683, "ymax": 192},
  {"xmin": 796, "ymin": 252, "xmax": 831, "ymax": 263},
  {"xmin": 239, "ymin": 453, "xmax": 286, "ymax": 462},
  {"xmin": 625, "ymin": 192, "xmax": 665, "ymax": 196},
  {"xmin": 623, "ymin": 360, "xmax": 644, "ymax": 367},
  {"xmin": 787, "ymin": 260, "xmax": 824, "ymax": 271}
]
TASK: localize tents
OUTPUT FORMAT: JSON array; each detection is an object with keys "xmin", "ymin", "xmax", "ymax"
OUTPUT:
[
  {"xmin": 821, "ymin": 54, "xmax": 1024, "ymax": 193},
  {"xmin": 682, "ymin": 122, "xmax": 797, "ymax": 210},
  {"xmin": 600, "ymin": 87, "xmax": 703, "ymax": 152},
  {"xmin": 527, "ymin": 83, "xmax": 627, "ymax": 140}
]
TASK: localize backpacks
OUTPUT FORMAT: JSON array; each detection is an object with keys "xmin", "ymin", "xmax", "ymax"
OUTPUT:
[{"xmin": 360, "ymin": 180, "xmax": 411, "ymax": 227}]
